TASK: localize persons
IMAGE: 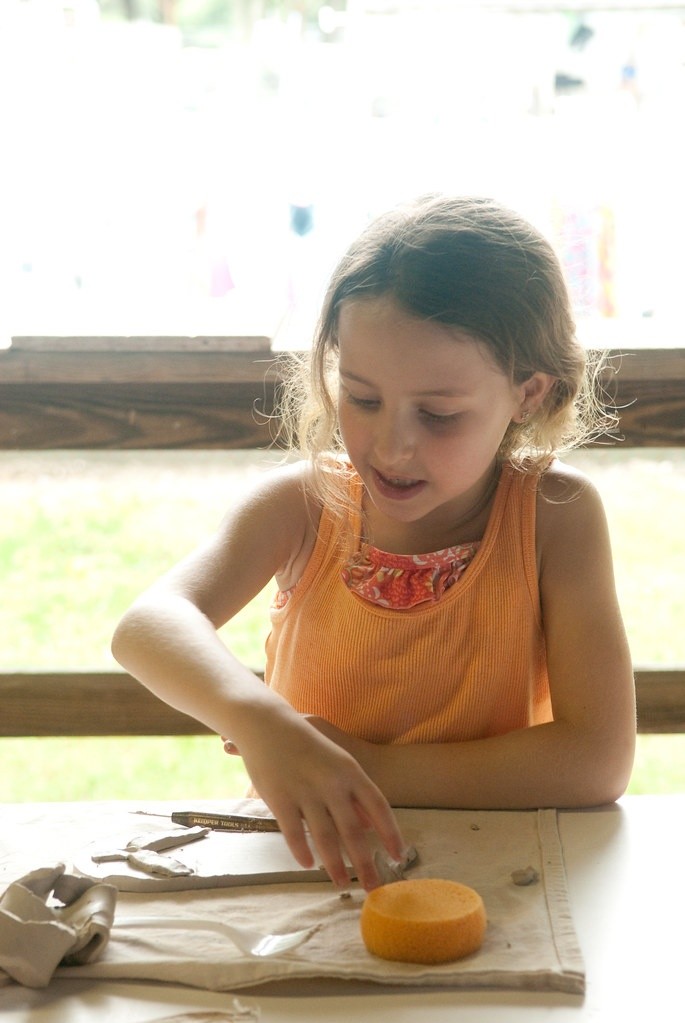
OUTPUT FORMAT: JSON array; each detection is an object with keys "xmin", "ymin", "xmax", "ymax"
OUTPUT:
[{"xmin": 111, "ymin": 197, "xmax": 637, "ymax": 893}]
